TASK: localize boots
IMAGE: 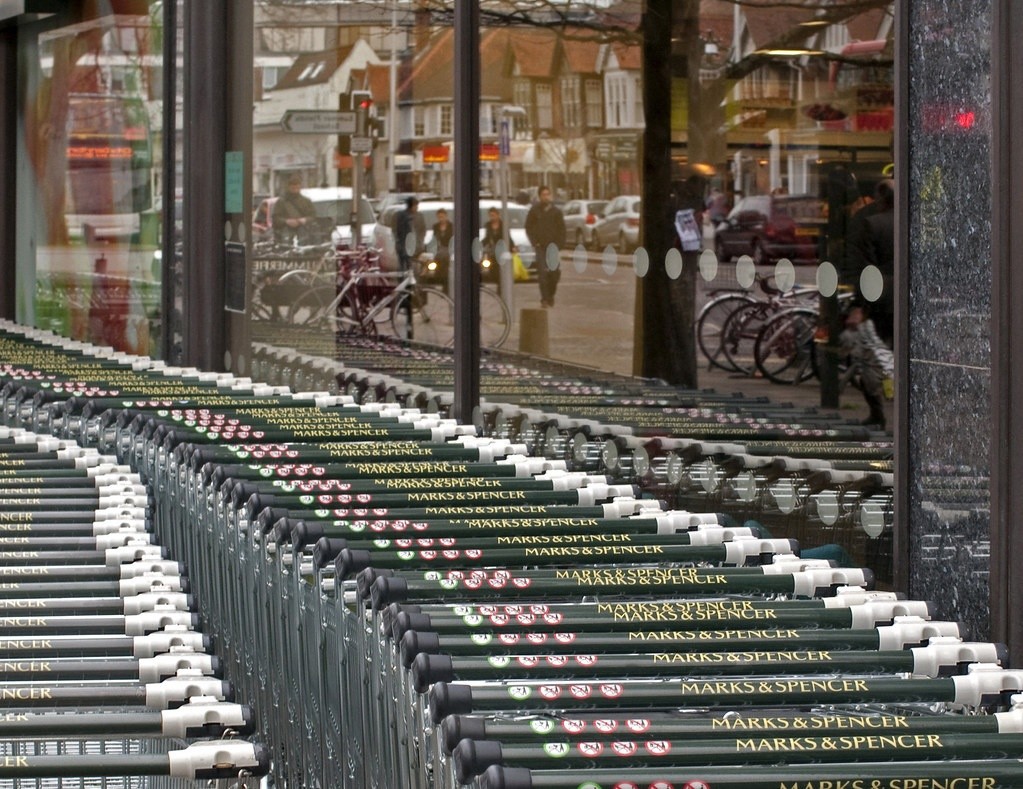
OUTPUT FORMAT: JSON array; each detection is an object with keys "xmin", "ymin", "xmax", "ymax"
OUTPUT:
[{"xmin": 859, "ymin": 392, "xmax": 887, "ymax": 431}]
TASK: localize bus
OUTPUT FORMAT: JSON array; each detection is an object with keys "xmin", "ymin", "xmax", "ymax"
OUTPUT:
[{"xmin": 31, "ymin": 90, "xmax": 157, "ymax": 270}]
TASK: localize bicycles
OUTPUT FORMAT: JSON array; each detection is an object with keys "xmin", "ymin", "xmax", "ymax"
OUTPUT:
[
  {"xmin": 698, "ymin": 271, "xmax": 865, "ymax": 391},
  {"xmin": 244, "ymin": 237, "xmax": 512, "ymax": 352}
]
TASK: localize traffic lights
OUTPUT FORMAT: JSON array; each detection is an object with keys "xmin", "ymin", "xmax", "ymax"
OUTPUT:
[{"xmin": 351, "ymin": 91, "xmax": 374, "ymax": 157}]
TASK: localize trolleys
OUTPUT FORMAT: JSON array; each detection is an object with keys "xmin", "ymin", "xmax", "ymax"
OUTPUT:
[
  {"xmin": 0, "ymin": 306, "xmax": 1023, "ymax": 789},
  {"xmin": 32, "ymin": 269, "xmax": 678, "ymax": 418}
]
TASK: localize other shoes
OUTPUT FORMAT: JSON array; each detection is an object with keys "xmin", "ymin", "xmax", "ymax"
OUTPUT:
[{"xmin": 542, "ymin": 295, "xmax": 555, "ymax": 307}]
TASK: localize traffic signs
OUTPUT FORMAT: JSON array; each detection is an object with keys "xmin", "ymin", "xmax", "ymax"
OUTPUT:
[{"xmin": 279, "ymin": 109, "xmax": 356, "ymax": 134}]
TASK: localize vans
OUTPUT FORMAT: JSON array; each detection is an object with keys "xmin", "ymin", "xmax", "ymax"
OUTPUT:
[{"xmin": 300, "ymin": 187, "xmax": 378, "ymax": 249}]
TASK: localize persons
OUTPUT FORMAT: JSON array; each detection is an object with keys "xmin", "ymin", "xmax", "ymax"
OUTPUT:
[
  {"xmin": 426, "ymin": 209, "xmax": 455, "ymax": 296},
  {"xmin": 847, "ymin": 180, "xmax": 895, "ymax": 350},
  {"xmin": 390, "ymin": 197, "xmax": 426, "ymax": 308},
  {"xmin": 816, "ymin": 163, "xmax": 888, "ymax": 432},
  {"xmin": 705, "ymin": 188, "xmax": 728, "ymax": 227},
  {"xmin": 525, "ymin": 186, "xmax": 566, "ymax": 308},
  {"xmin": 272, "ymin": 174, "xmax": 315, "ymax": 243},
  {"xmin": 480, "ymin": 207, "xmax": 519, "ymax": 297}
]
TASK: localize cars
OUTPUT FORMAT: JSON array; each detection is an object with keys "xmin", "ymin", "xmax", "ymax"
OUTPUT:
[
  {"xmin": 711, "ymin": 193, "xmax": 830, "ymax": 263},
  {"xmin": 591, "ymin": 195, "xmax": 641, "ymax": 253},
  {"xmin": 151, "ymin": 223, "xmax": 276, "ymax": 295},
  {"xmin": 562, "ymin": 199, "xmax": 611, "ymax": 250},
  {"xmin": 479, "ymin": 198, "xmax": 537, "ymax": 273},
  {"xmin": 252, "ymin": 198, "xmax": 279, "ymax": 235},
  {"xmin": 373, "ymin": 201, "xmax": 488, "ymax": 280}
]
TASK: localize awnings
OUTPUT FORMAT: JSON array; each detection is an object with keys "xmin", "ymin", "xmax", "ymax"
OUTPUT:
[{"xmin": 522, "ymin": 138, "xmax": 588, "ymax": 174}]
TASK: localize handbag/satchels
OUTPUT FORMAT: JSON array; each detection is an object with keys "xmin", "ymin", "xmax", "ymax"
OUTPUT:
[
  {"xmin": 512, "ymin": 252, "xmax": 530, "ymax": 280},
  {"xmin": 843, "ymin": 319, "xmax": 896, "ymax": 399}
]
